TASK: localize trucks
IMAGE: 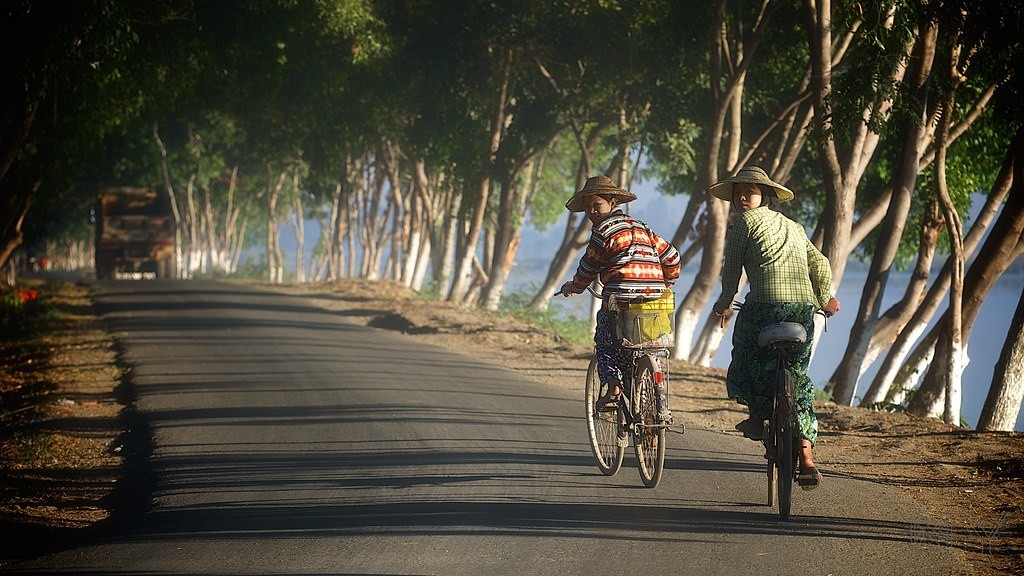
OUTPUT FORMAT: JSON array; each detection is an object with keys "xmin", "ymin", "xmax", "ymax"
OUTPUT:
[{"xmin": 89, "ymin": 187, "xmax": 178, "ymax": 280}]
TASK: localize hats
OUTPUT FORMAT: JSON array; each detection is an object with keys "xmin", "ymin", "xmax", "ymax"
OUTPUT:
[
  {"xmin": 565, "ymin": 176, "xmax": 637, "ymax": 212},
  {"xmin": 709, "ymin": 167, "xmax": 794, "ymax": 203}
]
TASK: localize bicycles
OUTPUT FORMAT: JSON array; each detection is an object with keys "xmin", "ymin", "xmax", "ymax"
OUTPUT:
[
  {"xmin": 553, "ymin": 278, "xmax": 686, "ymax": 489},
  {"xmin": 719, "ymin": 298, "xmax": 834, "ymax": 522}
]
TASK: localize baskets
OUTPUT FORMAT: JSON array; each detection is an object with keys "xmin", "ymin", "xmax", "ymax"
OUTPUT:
[{"xmin": 607, "ymin": 291, "xmax": 675, "ymax": 348}]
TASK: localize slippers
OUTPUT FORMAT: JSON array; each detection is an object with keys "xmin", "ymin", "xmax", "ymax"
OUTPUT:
[
  {"xmin": 596, "ymin": 395, "xmax": 619, "ymax": 413},
  {"xmin": 802, "ymin": 466, "xmax": 820, "ymax": 491},
  {"xmin": 735, "ymin": 420, "xmax": 762, "ymax": 442},
  {"xmin": 648, "ymin": 446, "xmax": 658, "ymax": 453}
]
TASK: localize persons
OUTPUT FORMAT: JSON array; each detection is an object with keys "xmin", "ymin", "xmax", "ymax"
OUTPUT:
[
  {"xmin": 560, "ymin": 175, "xmax": 682, "ymax": 450},
  {"xmin": 708, "ymin": 165, "xmax": 841, "ymax": 491}
]
